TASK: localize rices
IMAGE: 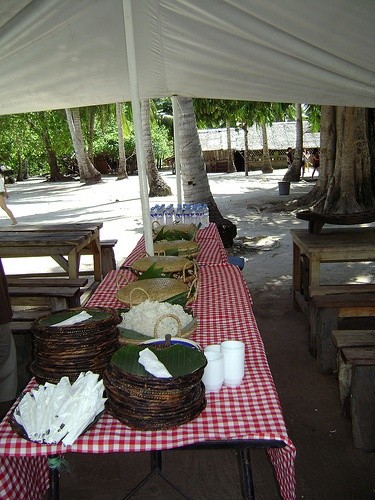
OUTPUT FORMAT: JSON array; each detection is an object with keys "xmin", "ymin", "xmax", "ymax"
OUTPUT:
[{"xmin": 116, "ymin": 299, "xmax": 193, "ymax": 339}]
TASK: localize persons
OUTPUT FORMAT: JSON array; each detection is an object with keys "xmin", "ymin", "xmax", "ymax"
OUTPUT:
[
  {"xmin": 0, "ymin": 174, "xmax": 17, "ymax": 225},
  {"xmin": 284, "ymin": 149, "xmax": 321, "ymax": 180},
  {"xmin": 0, "ymin": 260, "xmax": 17, "ymax": 421}
]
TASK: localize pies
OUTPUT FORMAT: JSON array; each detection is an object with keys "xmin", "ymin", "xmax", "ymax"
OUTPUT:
[
  {"xmin": 154, "ymin": 239, "xmax": 199, "ymax": 258},
  {"xmin": 155, "ymin": 222, "xmax": 191, "ymax": 234},
  {"xmin": 114, "ymin": 277, "xmax": 190, "ymax": 305}
]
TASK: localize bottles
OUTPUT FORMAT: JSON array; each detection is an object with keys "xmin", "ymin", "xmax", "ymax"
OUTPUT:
[{"xmin": 149, "ymin": 203, "xmax": 209, "ymax": 230}]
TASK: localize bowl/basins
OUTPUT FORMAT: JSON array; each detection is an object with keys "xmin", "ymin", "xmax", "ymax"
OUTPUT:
[{"xmin": 202, "ymin": 340, "xmax": 245, "ymax": 393}]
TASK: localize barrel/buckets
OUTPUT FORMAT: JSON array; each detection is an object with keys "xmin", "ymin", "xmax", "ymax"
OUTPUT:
[{"xmin": 278, "ymin": 182, "xmax": 290, "ymax": 195}]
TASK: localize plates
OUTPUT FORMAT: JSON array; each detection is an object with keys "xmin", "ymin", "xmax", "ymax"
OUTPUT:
[{"xmin": 136, "ymin": 337, "xmax": 202, "ymax": 353}]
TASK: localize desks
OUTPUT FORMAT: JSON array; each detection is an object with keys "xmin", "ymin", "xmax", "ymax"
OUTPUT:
[
  {"xmin": 0, "ymin": 222, "xmax": 104, "ymax": 307},
  {"xmin": 289, "ymin": 226, "xmax": 375, "ymax": 315}
]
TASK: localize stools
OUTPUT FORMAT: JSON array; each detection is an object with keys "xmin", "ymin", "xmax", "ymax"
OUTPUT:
[{"xmin": 228, "ymin": 256, "xmax": 244, "ymax": 271}]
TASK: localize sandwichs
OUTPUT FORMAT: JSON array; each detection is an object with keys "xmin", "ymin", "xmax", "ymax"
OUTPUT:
[{"xmin": 131, "ymin": 255, "xmax": 194, "ymax": 272}]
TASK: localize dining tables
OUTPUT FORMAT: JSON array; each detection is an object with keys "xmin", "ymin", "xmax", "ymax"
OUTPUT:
[
  {"xmin": 120, "ymin": 222, "xmax": 229, "ymax": 268},
  {"xmin": 0, "ymin": 264, "xmax": 296, "ymax": 500}
]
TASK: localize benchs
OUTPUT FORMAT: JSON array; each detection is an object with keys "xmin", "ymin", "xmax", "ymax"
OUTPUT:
[
  {"xmin": 309, "ymin": 285, "xmax": 375, "ymax": 450},
  {"xmin": 6, "ymin": 277, "xmax": 88, "ymax": 364},
  {"xmin": 100, "ymin": 239, "xmax": 116, "ymax": 279}
]
